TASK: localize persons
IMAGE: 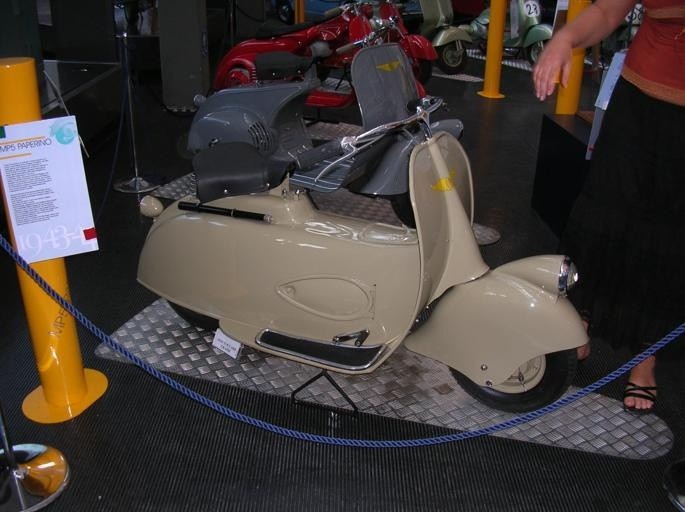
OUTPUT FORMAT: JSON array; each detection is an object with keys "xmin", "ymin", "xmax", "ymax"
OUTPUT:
[{"xmin": 533, "ymin": 0, "xmax": 685, "ymax": 414}]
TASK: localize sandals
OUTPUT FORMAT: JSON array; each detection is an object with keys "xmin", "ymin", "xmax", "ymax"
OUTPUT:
[{"xmin": 623, "ymin": 344, "xmax": 659, "ymax": 414}]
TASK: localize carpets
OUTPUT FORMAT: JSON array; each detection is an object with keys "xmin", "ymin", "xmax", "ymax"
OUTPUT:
[
  {"xmin": 93, "ymin": 299, "xmax": 675, "ymax": 463},
  {"xmin": 304, "ymin": 120, "xmax": 364, "ymax": 140},
  {"xmin": 318, "ymin": 80, "xmax": 354, "ymax": 96},
  {"xmin": 149, "ymin": 173, "xmax": 502, "ymax": 246},
  {"xmin": 431, "ymin": 47, "xmax": 610, "ymax": 83}
]
